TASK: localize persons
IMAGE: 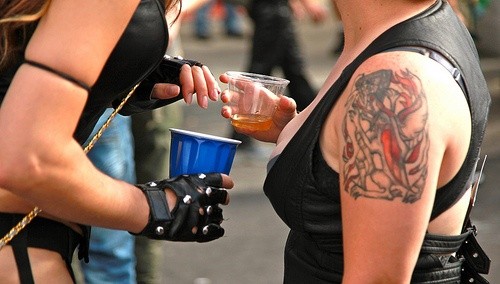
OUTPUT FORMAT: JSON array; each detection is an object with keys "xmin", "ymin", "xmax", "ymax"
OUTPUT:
[
  {"xmin": 223, "ymin": 0, "xmax": 328, "ymax": 151},
  {"xmin": 78, "ymin": 0, "xmax": 207, "ymax": 284},
  {"xmin": 0, "ymin": 0, "xmax": 233, "ymax": 284},
  {"xmin": 219, "ymin": 1, "xmax": 492, "ymax": 284}
]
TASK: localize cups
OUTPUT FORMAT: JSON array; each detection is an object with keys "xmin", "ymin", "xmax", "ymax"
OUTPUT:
[
  {"xmin": 224, "ymin": 70, "xmax": 290, "ymax": 130},
  {"xmin": 168, "ymin": 127, "xmax": 242, "ymax": 179}
]
